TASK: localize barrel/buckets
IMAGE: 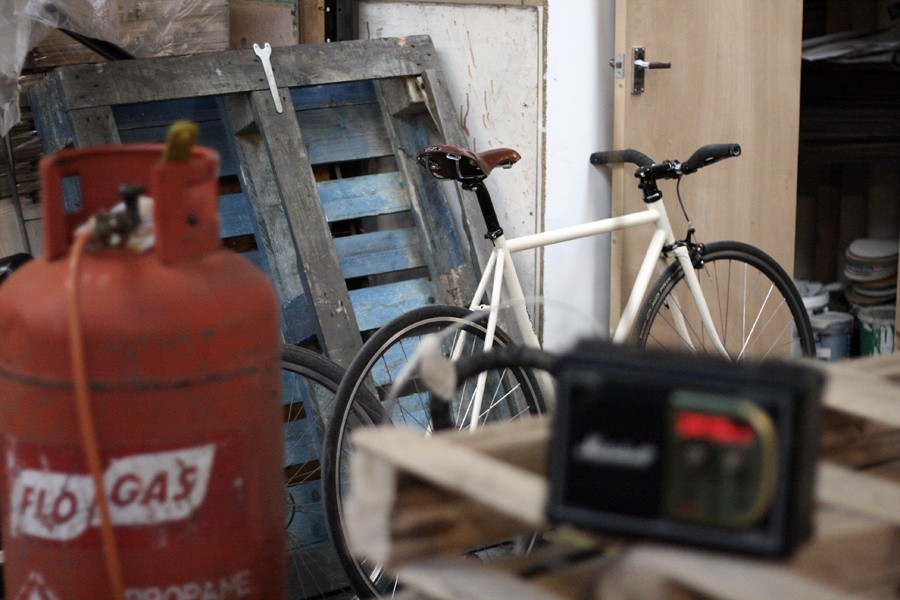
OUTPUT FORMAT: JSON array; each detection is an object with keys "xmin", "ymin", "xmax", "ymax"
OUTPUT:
[
  {"xmin": 792, "ymin": 280, "xmax": 830, "ymax": 355},
  {"xmin": 812, "ymin": 312, "xmax": 854, "ymax": 361},
  {"xmin": 858, "ymin": 305, "xmax": 896, "ymax": 357}
]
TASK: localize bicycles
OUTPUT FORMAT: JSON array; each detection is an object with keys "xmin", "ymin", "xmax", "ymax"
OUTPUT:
[{"xmin": 276, "ymin": 143, "xmax": 819, "ymax": 600}]
